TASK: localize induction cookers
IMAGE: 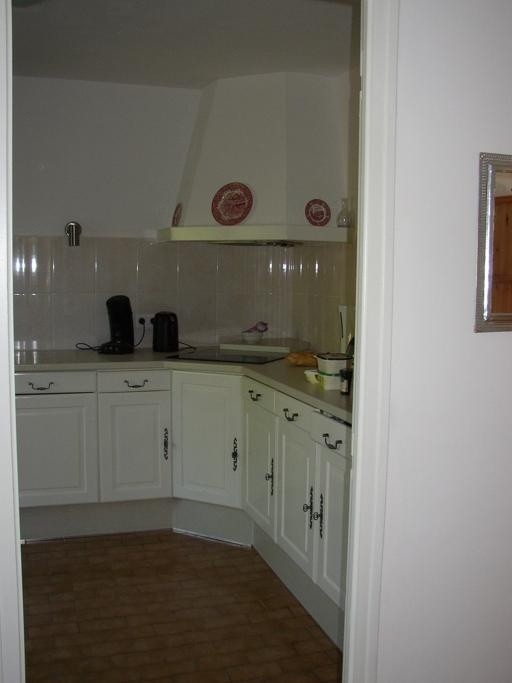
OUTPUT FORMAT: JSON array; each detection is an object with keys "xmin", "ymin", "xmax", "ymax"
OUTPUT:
[{"xmin": 166, "ymin": 349, "xmax": 290, "ymax": 365}]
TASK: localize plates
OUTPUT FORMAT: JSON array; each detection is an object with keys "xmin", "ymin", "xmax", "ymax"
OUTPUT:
[
  {"xmin": 304, "ymin": 198, "xmax": 331, "ymax": 226},
  {"xmin": 211, "ymin": 181, "xmax": 253, "ymax": 225},
  {"xmin": 172, "ymin": 202, "xmax": 183, "ymax": 226}
]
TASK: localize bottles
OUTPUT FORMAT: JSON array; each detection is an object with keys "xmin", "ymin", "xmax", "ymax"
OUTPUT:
[
  {"xmin": 340, "ymin": 368, "xmax": 353, "ymax": 396},
  {"xmin": 336, "ymin": 199, "xmax": 352, "ymax": 227}
]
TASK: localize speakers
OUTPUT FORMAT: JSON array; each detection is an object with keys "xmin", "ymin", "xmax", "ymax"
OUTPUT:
[{"xmin": 106, "ymin": 294, "xmax": 135, "ymax": 354}]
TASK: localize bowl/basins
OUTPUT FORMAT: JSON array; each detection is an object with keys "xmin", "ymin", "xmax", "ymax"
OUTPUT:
[
  {"xmin": 304, "ymin": 370, "xmax": 321, "ymax": 385},
  {"xmin": 241, "ymin": 332, "xmax": 261, "ymax": 344}
]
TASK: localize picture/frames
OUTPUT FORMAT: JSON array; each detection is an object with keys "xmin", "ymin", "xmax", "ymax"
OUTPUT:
[{"xmin": 475, "ymin": 153, "xmax": 512, "ymax": 333}]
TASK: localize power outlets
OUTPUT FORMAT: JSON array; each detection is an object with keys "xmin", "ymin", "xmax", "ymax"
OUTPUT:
[{"xmin": 136, "ymin": 314, "xmax": 154, "ymax": 328}]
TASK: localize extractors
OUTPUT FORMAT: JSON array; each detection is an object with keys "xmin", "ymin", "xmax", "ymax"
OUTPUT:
[{"xmin": 157, "ymin": 225, "xmax": 348, "ymax": 247}]
